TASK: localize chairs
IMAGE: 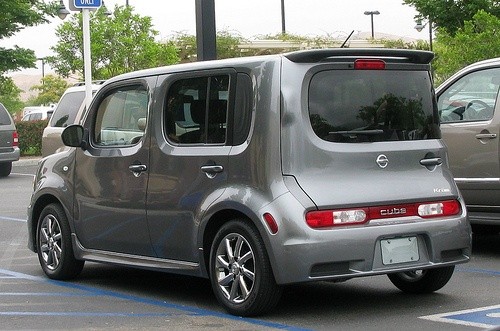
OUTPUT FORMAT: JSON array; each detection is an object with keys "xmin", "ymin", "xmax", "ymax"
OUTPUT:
[{"xmin": 178, "ymin": 99, "xmax": 227, "ymax": 144}]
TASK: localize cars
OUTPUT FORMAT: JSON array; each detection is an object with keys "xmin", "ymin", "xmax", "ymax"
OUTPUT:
[{"xmin": 24, "ymin": 46, "xmax": 474, "ymax": 317}]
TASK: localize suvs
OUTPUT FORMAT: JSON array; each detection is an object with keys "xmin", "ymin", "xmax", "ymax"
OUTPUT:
[
  {"xmin": 19, "ymin": 106, "xmax": 57, "ymax": 121},
  {"xmin": 0, "ymin": 101, "xmax": 21, "ymax": 178},
  {"xmin": 38, "ymin": 78, "xmax": 148, "ymax": 158},
  {"xmin": 435, "ymin": 57, "xmax": 500, "ymax": 227}
]
